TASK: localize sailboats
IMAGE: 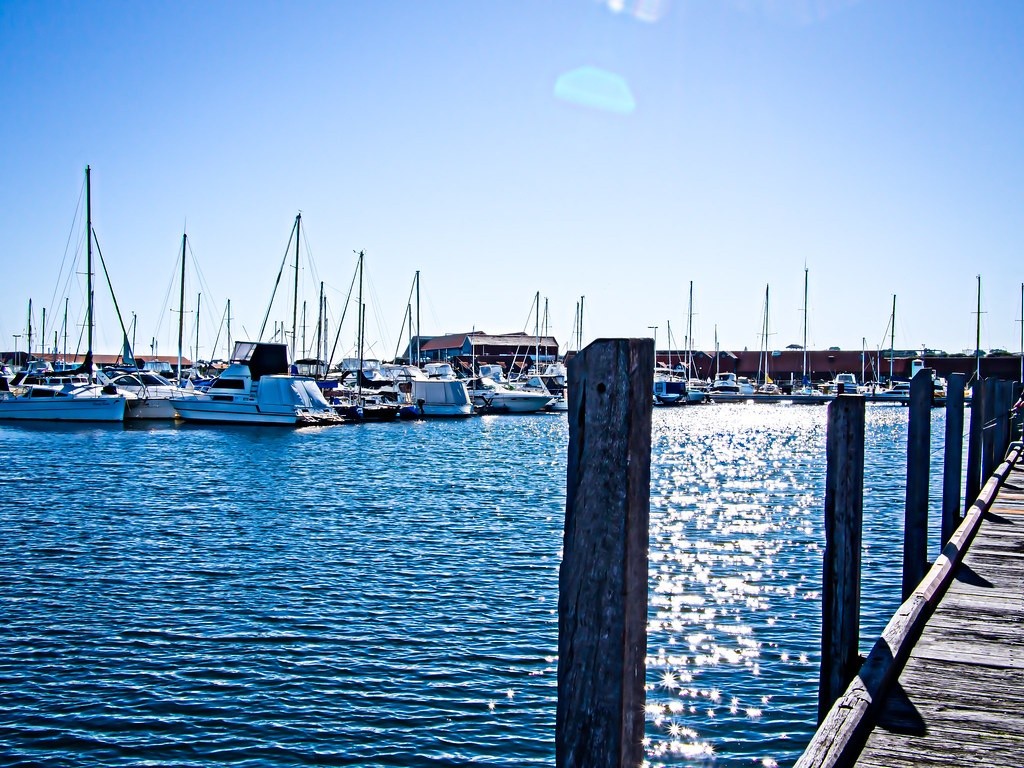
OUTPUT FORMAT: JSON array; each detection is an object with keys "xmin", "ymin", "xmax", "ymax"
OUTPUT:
[
  {"xmin": 792, "ymin": 268, "xmax": 823, "ymax": 404},
  {"xmin": 289, "ymin": 251, "xmax": 757, "ymax": 421},
  {"xmin": 753, "ymin": 283, "xmax": 784, "ymax": 404},
  {"xmin": 168, "ymin": 213, "xmax": 345, "ymax": 426},
  {"xmin": 1, "ymin": 165, "xmax": 218, "ymax": 421}
]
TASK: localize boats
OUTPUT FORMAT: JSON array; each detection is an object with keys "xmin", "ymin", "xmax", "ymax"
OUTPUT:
[{"xmin": 817, "ymin": 374, "xmax": 859, "ymax": 394}]
